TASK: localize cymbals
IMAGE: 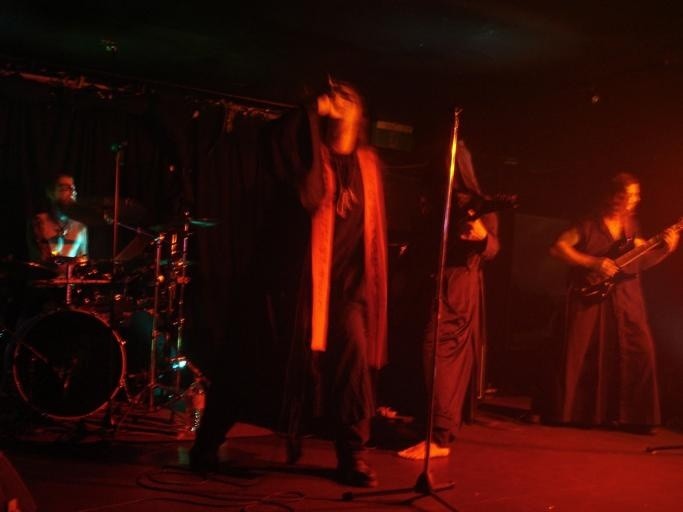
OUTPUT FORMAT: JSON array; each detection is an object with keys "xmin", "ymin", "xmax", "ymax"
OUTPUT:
[{"xmin": 0, "ymin": 255, "xmax": 53, "ymax": 272}]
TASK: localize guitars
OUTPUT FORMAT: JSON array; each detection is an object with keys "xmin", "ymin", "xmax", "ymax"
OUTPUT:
[{"xmin": 566, "ymin": 219, "xmax": 683, "ymax": 304}]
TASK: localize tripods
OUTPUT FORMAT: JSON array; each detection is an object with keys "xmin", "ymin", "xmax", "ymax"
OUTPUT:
[
  {"xmin": 341, "ymin": 107, "xmax": 460, "ymax": 512},
  {"xmin": 110, "ymin": 208, "xmax": 212, "ymax": 433}
]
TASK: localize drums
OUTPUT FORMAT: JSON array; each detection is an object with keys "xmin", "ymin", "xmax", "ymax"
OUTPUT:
[
  {"xmin": 112, "ymin": 263, "xmax": 181, "ymax": 311},
  {"xmin": 12, "ymin": 304, "xmax": 128, "ymax": 423},
  {"xmin": 24, "ymin": 274, "xmax": 113, "ymax": 326}
]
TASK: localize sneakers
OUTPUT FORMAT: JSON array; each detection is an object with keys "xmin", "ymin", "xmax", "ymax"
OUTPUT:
[
  {"xmin": 336, "ymin": 459, "xmax": 378, "ymax": 487},
  {"xmin": 187, "ymin": 445, "xmax": 219, "ymax": 476}
]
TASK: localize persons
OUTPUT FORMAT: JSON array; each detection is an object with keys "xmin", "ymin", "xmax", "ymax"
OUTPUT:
[
  {"xmin": 547, "ymin": 173, "xmax": 678, "ymax": 435},
  {"xmin": 179, "ymin": 106, "xmax": 284, "ymax": 480},
  {"xmin": 21, "ymin": 161, "xmax": 88, "ymax": 306},
  {"xmin": 292, "ymin": 87, "xmax": 393, "ymax": 481},
  {"xmin": 396, "ymin": 136, "xmax": 501, "ymax": 459}
]
